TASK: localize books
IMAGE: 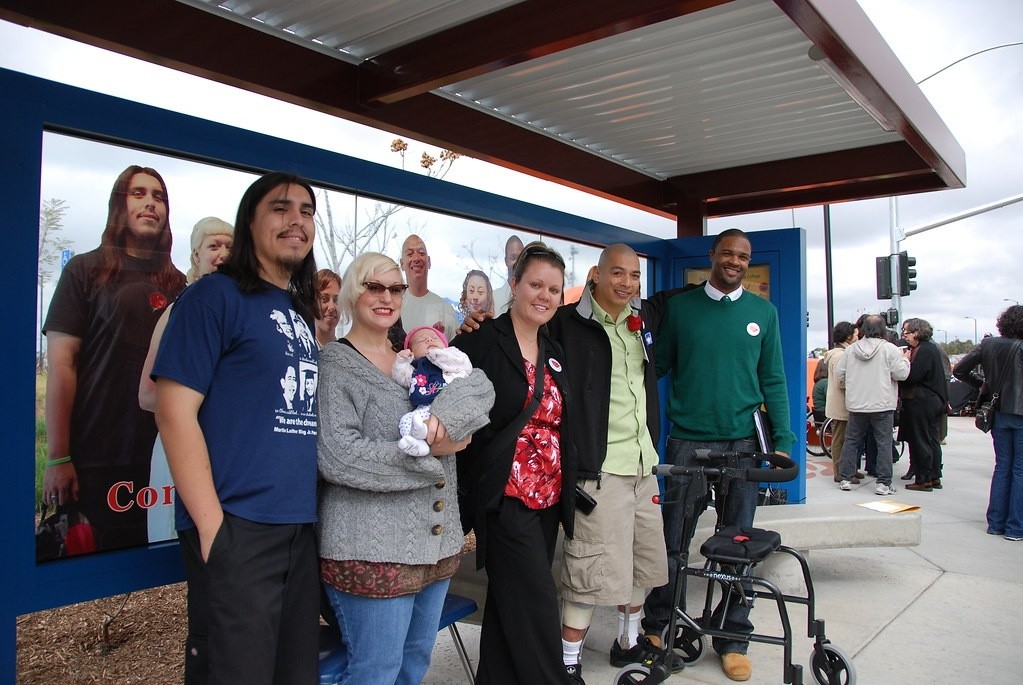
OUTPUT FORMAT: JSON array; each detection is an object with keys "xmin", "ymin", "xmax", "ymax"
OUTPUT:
[{"xmin": 752, "ymin": 409, "xmax": 776, "ymax": 465}]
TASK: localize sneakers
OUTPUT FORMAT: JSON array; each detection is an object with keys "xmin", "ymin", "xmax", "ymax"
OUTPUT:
[
  {"xmin": 564, "ymin": 664, "xmax": 585, "ymax": 685},
  {"xmin": 839, "ymin": 480, "xmax": 851, "ymax": 490},
  {"xmin": 720, "ymin": 652, "xmax": 752, "ymax": 681},
  {"xmin": 610, "ymin": 633, "xmax": 684, "ymax": 671},
  {"xmin": 875, "ymin": 483, "xmax": 899, "ymax": 495},
  {"xmin": 646, "ymin": 635, "xmax": 661, "ymax": 647}
]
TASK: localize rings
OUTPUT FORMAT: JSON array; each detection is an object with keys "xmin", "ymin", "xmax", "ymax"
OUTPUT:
[{"xmin": 51, "ymin": 496, "xmax": 56, "ymax": 498}]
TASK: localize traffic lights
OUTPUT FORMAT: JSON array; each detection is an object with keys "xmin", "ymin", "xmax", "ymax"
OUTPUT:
[
  {"xmin": 806, "ymin": 312, "xmax": 810, "ymax": 327},
  {"xmin": 899, "ymin": 251, "xmax": 917, "ymax": 297}
]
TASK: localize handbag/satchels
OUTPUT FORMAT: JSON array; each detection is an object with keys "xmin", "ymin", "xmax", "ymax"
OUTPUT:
[
  {"xmin": 975, "ymin": 401, "xmax": 996, "ymax": 433},
  {"xmin": 456, "ymin": 450, "xmax": 475, "ymax": 534},
  {"xmin": 36, "ymin": 497, "xmax": 95, "ymax": 562}
]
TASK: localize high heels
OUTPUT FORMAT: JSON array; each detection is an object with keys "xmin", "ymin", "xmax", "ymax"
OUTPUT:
[{"xmin": 901, "ymin": 467, "xmax": 916, "ymax": 479}]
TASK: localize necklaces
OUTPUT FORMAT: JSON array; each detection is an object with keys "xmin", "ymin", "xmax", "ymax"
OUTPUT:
[{"xmin": 515, "ymin": 327, "xmax": 535, "ymax": 347}]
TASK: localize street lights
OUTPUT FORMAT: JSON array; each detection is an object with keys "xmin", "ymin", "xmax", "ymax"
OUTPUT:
[
  {"xmin": 965, "ymin": 317, "xmax": 977, "ymax": 344},
  {"xmin": 937, "ymin": 330, "xmax": 947, "ymax": 343},
  {"xmin": 1004, "ymin": 299, "xmax": 1018, "ymax": 305}
]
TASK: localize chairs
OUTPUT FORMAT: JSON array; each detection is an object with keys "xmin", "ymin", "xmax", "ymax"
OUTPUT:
[{"xmin": 319, "ymin": 583, "xmax": 477, "ymax": 685}]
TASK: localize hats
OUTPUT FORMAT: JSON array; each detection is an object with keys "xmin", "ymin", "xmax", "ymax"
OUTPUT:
[{"xmin": 404, "ymin": 327, "xmax": 448, "ymax": 350}]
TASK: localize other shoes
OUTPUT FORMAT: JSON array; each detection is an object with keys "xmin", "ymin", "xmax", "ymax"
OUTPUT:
[
  {"xmin": 987, "ymin": 526, "xmax": 1005, "ymax": 535},
  {"xmin": 834, "ymin": 476, "xmax": 860, "ymax": 484},
  {"xmin": 854, "ymin": 472, "xmax": 865, "ymax": 479},
  {"xmin": 932, "ymin": 479, "xmax": 942, "ymax": 488},
  {"xmin": 939, "ymin": 439, "xmax": 946, "ymax": 445},
  {"xmin": 1005, "ymin": 535, "xmax": 1023, "ymax": 541},
  {"xmin": 906, "ymin": 481, "xmax": 933, "ymax": 491}
]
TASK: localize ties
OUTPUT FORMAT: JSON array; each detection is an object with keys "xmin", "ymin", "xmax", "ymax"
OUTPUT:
[{"xmin": 720, "ymin": 296, "xmax": 731, "ymax": 302}]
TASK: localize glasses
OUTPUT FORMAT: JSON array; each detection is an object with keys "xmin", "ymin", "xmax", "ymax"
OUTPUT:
[
  {"xmin": 522, "ymin": 247, "xmax": 565, "ymax": 268},
  {"xmin": 901, "ymin": 328, "xmax": 908, "ymax": 331},
  {"xmin": 903, "ymin": 332, "xmax": 914, "ymax": 337},
  {"xmin": 363, "ymin": 282, "xmax": 409, "ymax": 297}
]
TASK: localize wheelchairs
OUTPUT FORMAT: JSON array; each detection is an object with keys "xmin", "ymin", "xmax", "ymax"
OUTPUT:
[{"xmin": 807, "ymin": 412, "xmax": 835, "ymax": 459}]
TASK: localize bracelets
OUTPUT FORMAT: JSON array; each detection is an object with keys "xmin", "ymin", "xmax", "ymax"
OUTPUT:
[{"xmin": 45, "ymin": 456, "xmax": 71, "ymax": 466}]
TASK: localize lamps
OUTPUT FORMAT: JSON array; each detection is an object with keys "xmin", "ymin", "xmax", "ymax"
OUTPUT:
[{"xmin": 809, "ymin": 44, "xmax": 896, "ymax": 132}]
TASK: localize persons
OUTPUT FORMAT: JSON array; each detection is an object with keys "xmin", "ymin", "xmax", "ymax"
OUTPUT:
[
  {"xmin": 826, "ymin": 314, "xmax": 949, "ymax": 494},
  {"xmin": 813, "ymin": 359, "xmax": 828, "ymax": 410},
  {"xmin": 953, "ymin": 305, "xmax": 1023, "ymax": 540},
  {"xmin": 398, "ymin": 234, "xmax": 458, "ymax": 343},
  {"xmin": 458, "ymin": 270, "xmax": 494, "ymax": 317},
  {"xmin": 150, "ymin": 172, "xmax": 319, "ymax": 685},
  {"xmin": 493, "ymin": 235, "xmax": 525, "ymax": 319},
  {"xmin": 391, "ymin": 326, "xmax": 473, "ymax": 457},
  {"xmin": 137, "ymin": 217, "xmax": 235, "ymax": 543},
  {"xmin": 455, "ymin": 243, "xmax": 706, "ymax": 685},
  {"xmin": 449, "ymin": 240, "xmax": 579, "ymax": 685},
  {"xmin": 316, "ymin": 252, "xmax": 473, "ymax": 685},
  {"xmin": 641, "ymin": 229, "xmax": 798, "ymax": 680},
  {"xmin": 314, "ymin": 269, "xmax": 342, "ymax": 351},
  {"xmin": 41, "ymin": 166, "xmax": 187, "ymax": 557}
]
